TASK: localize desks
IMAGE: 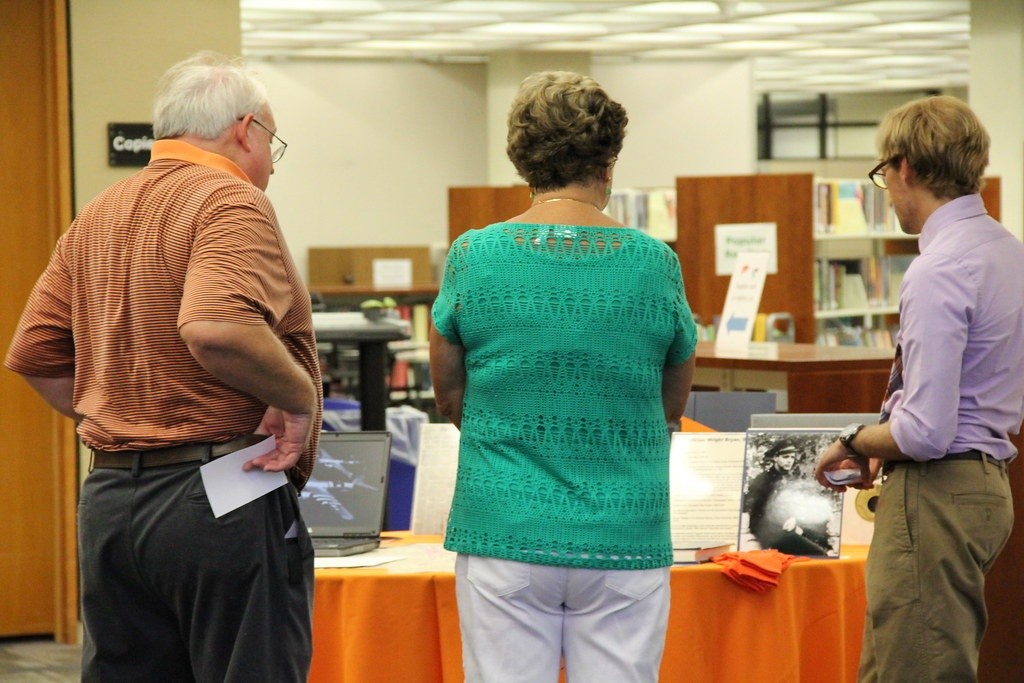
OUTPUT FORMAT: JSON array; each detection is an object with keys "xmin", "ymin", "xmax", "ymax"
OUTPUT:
[
  {"xmin": 303, "ymin": 526, "xmax": 870, "ymax": 683},
  {"xmin": 309, "ymin": 310, "xmax": 412, "ymax": 432}
]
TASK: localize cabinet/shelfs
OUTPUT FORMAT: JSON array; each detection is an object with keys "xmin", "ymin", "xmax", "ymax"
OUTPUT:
[
  {"xmin": 310, "ymin": 288, "xmax": 441, "ymax": 403},
  {"xmin": 448, "ymin": 174, "xmax": 1000, "ymax": 346}
]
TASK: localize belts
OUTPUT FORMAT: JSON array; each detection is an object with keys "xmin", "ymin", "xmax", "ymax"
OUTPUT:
[
  {"xmin": 93, "ymin": 437, "xmax": 270, "ymax": 469},
  {"xmin": 941, "ymin": 450, "xmax": 1007, "ymax": 470}
]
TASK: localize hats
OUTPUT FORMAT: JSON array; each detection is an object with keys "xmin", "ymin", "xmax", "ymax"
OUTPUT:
[{"xmin": 768, "ymin": 438, "xmax": 798, "ymax": 457}]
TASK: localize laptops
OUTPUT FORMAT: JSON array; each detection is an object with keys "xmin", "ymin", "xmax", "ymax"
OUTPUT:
[{"xmin": 297, "ymin": 431, "xmax": 392, "ymax": 556}]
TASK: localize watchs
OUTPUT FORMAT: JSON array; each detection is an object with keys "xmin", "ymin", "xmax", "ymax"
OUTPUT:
[{"xmin": 839, "ymin": 422, "xmax": 866, "ymax": 459}]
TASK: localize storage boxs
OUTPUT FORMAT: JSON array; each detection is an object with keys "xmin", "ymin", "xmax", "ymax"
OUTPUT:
[{"xmin": 308, "ymin": 246, "xmax": 433, "ymax": 293}]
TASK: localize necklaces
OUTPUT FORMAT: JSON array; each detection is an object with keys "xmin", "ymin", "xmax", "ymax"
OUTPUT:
[{"xmin": 531, "ymin": 198, "xmax": 599, "ymax": 208}]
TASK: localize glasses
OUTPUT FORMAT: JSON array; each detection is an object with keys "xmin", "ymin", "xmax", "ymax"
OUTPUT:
[
  {"xmin": 237, "ymin": 116, "xmax": 287, "ymax": 162},
  {"xmin": 868, "ymin": 160, "xmax": 894, "ymax": 189}
]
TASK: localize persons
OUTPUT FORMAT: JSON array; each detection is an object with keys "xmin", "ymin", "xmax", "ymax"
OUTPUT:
[
  {"xmin": 749, "ymin": 439, "xmax": 838, "ymax": 558},
  {"xmin": 3, "ymin": 55, "xmax": 324, "ymax": 683},
  {"xmin": 429, "ymin": 70, "xmax": 698, "ymax": 683},
  {"xmin": 814, "ymin": 95, "xmax": 1024, "ymax": 683}
]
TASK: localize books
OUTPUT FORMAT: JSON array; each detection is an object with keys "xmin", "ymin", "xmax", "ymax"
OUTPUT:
[
  {"xmin": 604, "ymin": 190, "xmax": 677, "ymax": 238},
  {"xmin": 672, "ymin": 541, "xmax": 735, "ymax": 564},
  {"xmin": 812, "ymin": 176, "xmax": 901, "ymax": 348},
  {"xmin": 387, "ymin": 304, "xmax": 434, "ymax": 400}
]
